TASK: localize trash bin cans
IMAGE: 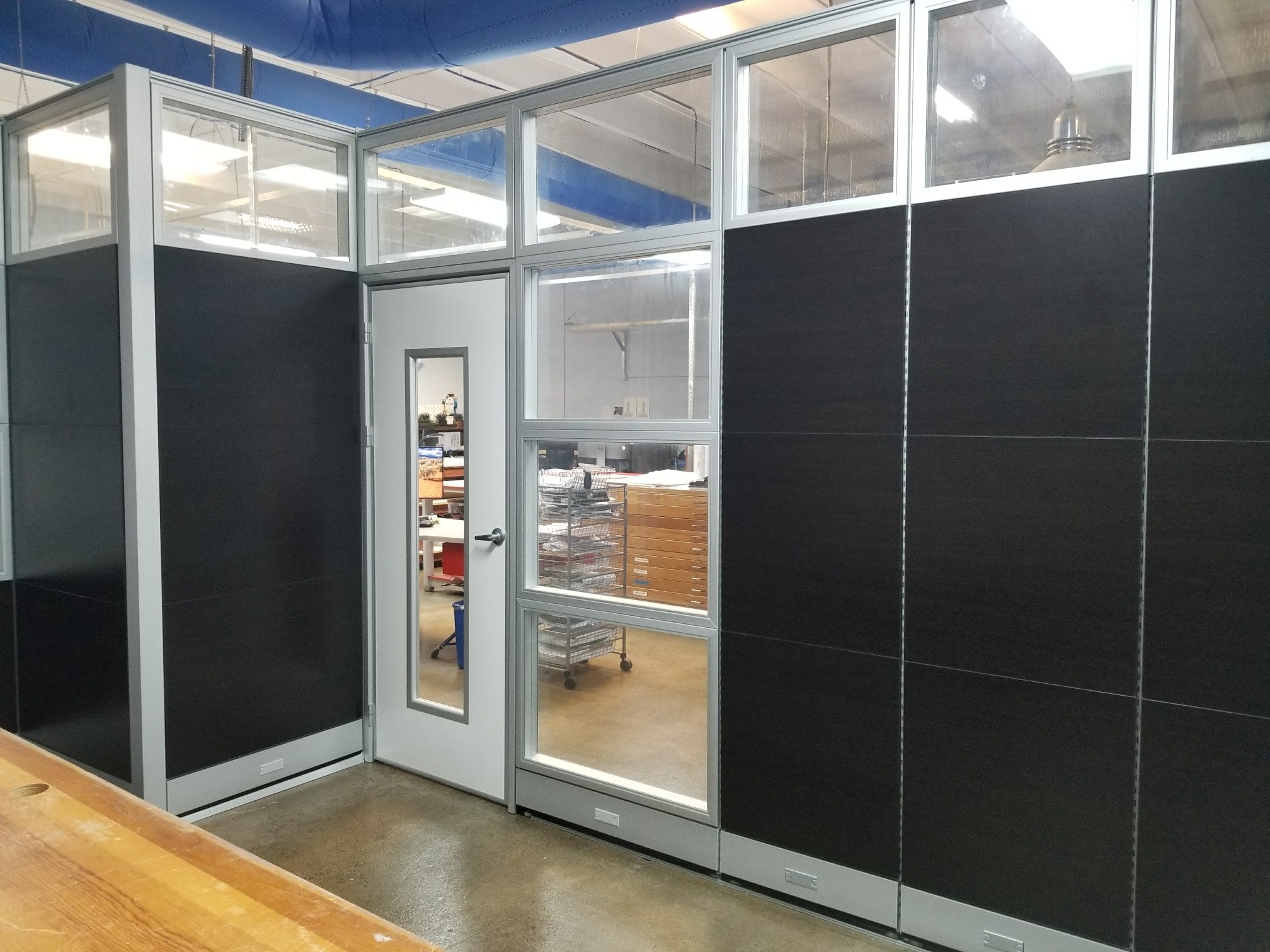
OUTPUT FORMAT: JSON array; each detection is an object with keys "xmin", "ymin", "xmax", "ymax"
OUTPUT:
[{"xmin": 452, "ymin": 599, "xmax": 464, "ymax": 668}]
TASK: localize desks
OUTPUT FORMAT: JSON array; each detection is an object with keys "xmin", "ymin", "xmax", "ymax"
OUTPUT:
[{"xmin": 418, "ymin": 479, "xmax": 465, "ymax": 591}]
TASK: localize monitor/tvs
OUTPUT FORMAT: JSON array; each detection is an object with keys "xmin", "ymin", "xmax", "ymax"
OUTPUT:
[{"xmin": 418, "ymin": 446, "xmax": 444, "ymax": 499}]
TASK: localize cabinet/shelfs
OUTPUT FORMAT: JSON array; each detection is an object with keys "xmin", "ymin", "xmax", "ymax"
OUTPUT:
[{"xmin": 536, "ymin": 466, "xmax": 633, "ymax": 690}]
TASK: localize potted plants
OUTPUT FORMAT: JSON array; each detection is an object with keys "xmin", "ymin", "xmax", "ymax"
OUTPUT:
[{"xmin": 418, "ymin": 412, "xmax": 464, "ymax": 427}]
